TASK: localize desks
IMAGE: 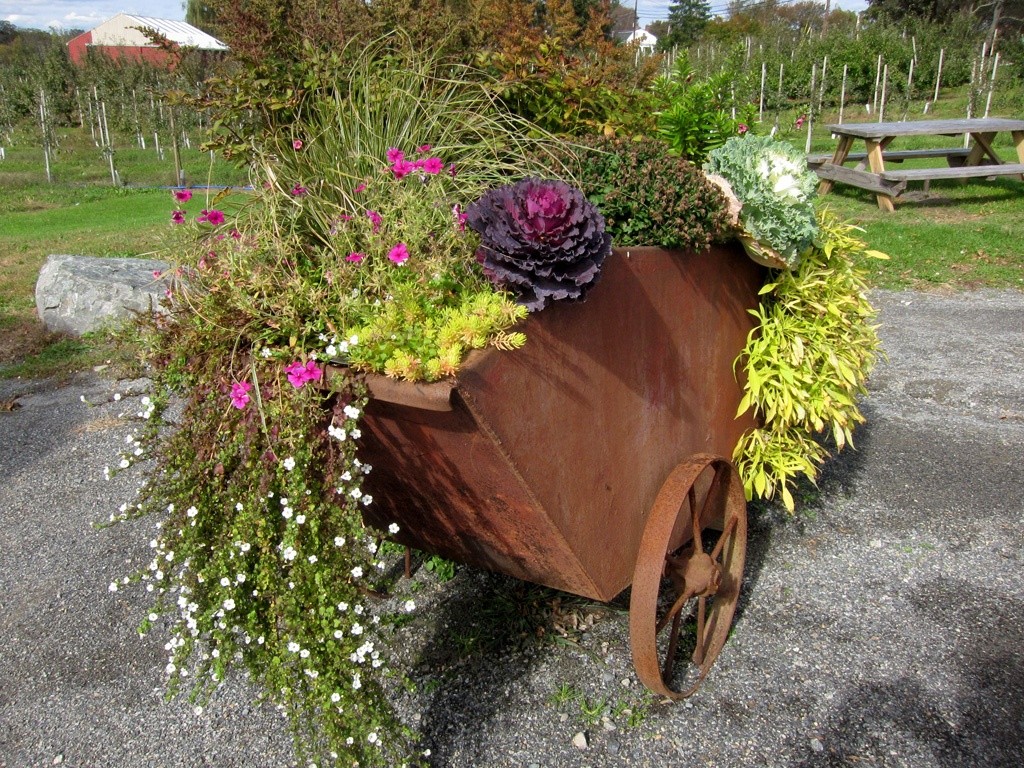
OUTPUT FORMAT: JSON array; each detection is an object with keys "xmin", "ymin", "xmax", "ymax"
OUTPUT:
[{"xmin": 817, "ymin": 117, "xmax": 1024, "ymax": 213}]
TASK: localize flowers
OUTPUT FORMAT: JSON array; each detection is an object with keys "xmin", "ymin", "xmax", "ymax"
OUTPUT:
[{"xmin": 75, "ymin": 130, "xmax": 819, "ymax": 768}]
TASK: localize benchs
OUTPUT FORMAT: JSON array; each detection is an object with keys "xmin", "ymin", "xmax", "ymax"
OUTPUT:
[
  {"xmin": 805, "ymin": 148, "xmax": 972, "ymax": 180},
  {"xmin": 878, "ymin": 163, "xmax": 1024, "ymax": 211}
]
